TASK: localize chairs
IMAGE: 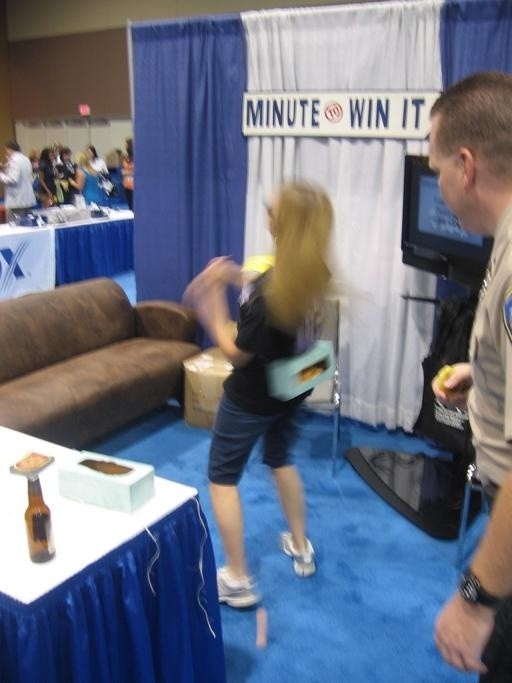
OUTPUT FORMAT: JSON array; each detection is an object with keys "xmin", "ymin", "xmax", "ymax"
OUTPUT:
[
  {"xmin": 455, "ymin": 448, "xmax": 497, "ymax": 579},
  {"xmin": 307, "ymin": 297, "xmax": 346, "ymax": 476}
]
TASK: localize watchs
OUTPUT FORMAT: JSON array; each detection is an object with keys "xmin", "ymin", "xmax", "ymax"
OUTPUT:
[{"xmin": 456, "ymin": 566, "xmax": 504, "ymax": 610}]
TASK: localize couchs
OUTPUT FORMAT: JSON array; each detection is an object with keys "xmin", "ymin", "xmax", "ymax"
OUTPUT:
[{"xmin": 0, "ymin": 273, "xmax": 205, "ymax": 445}]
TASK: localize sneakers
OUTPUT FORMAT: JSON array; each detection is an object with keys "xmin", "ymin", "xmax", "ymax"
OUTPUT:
[
  {"xmin": 282, "ymin": 533, "xmax": 316, "ymax": 577},
  {"xmin": 216, "ymin": 567, "xmax": 256, "ymax": 607}
]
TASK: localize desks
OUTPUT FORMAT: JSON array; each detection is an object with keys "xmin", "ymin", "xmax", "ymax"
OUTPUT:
[
  {"xmin": 0, "ymin": 421, "xmax": 229, "ymax": 681},
  {"xmin": 0, "ymin": 204, "xmax": 135, "ymax": 299}
]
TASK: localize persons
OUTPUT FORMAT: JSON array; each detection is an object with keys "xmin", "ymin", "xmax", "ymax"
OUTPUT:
[
  {"xmin": 427, "ymin": 74, "xmax": 512, "ymax": 683},
  {"xmin": 0, "ymin": 136, "xmax": 134, "ymax": 224},
  {"xmin": 179, "ymin": 180, "xmax": 333, "ymax": 609}
]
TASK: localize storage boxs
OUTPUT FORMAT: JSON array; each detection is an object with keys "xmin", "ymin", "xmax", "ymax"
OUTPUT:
[{"xmin": 183, "ymin": 345, "xmax": 233, "ymax": 429}]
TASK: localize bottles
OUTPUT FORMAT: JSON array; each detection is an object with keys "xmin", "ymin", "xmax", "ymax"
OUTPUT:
[{"xmin": 22, "ymin": 472, "xmax": 57, "ymax": 564}]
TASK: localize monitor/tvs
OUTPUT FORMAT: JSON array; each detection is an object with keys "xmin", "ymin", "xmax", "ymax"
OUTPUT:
[{"xmin": 401, "ymin": 154, "xmax": 494, "ymax": 288}]
